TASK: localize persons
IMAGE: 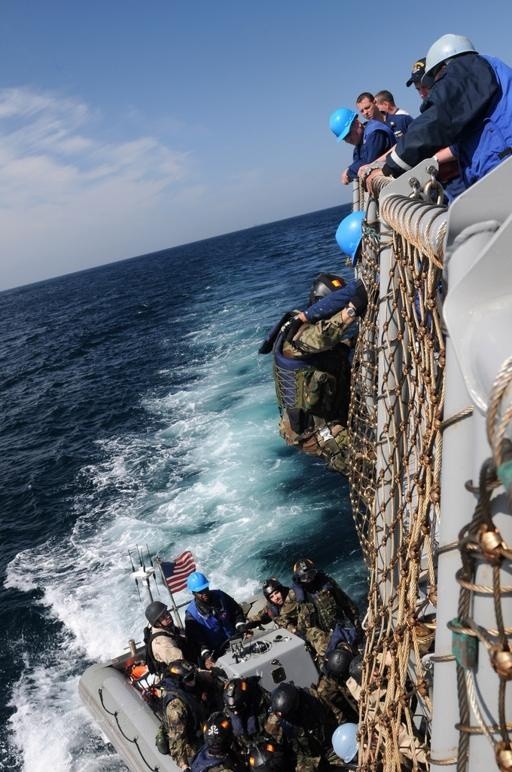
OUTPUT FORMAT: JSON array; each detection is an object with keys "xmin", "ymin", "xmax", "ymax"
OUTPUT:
[
  {"xmin": 273, "ymin": 272, "xmax": 375, "ymax": 496},
  {"xmin": 141, "ymin": 559, "xmax": 362, "ymax": 770},
  {"xmin": 326, "ymin": 31, "xmax": 512, "ymax": 210},
  {"xmin": 295, "ymin": 210, "xmax": 367, "ymax": 332}
]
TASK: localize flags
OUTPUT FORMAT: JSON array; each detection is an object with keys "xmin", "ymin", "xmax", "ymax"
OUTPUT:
[{"xmin": 161, "ymin": 551, "xmax": 197, "ymax": 594}]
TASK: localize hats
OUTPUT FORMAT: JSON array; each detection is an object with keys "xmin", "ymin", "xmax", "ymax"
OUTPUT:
[{"xmin": 406, "ymin": 57, "xmax": 426, "ymax": 87}]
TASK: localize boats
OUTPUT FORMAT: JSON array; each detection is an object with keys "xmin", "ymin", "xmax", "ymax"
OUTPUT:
[{"xmin": 74, "ymin": 543, "xmax": 321, "ymax": 772}]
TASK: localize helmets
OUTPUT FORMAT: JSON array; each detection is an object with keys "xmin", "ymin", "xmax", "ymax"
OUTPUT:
[
  {"xmin": 420, "ymin": 34, "xmax": 479, "ymax": 87},
  {"xmin": 331, "ymin": 723, "xmax": 359, "ymax": 764},
  {"xmin": 335, "ymin": 211, "xmax": 365, "ymax": 267},
  {"xmin": 309, "ymin": 273, "xmax": 345, "ymax": 307},
  {"xmin": 168, "ymin": 659, "xmax": 197, "ymax": 687},
  {"xmin": 263, "ymin": 579, "xmax": 284, "ymax": 603},
  {"xmin": 145, "ymin": 601, "xmax": 167, "ymax": 626},
  {"xmin": 187, "ymin": 572, "xmax": 210, "ymax": 592},
  {"xmin": 293, "ymin": 559, "xmax": 317, "ymax": 582},
  {"xmin": 203, "ymin": 678, "xmax": 299, "ymax": 771},
  {"xmin": 329, "ymin": 108, "xmax": 357, "ymax": 142}
]
